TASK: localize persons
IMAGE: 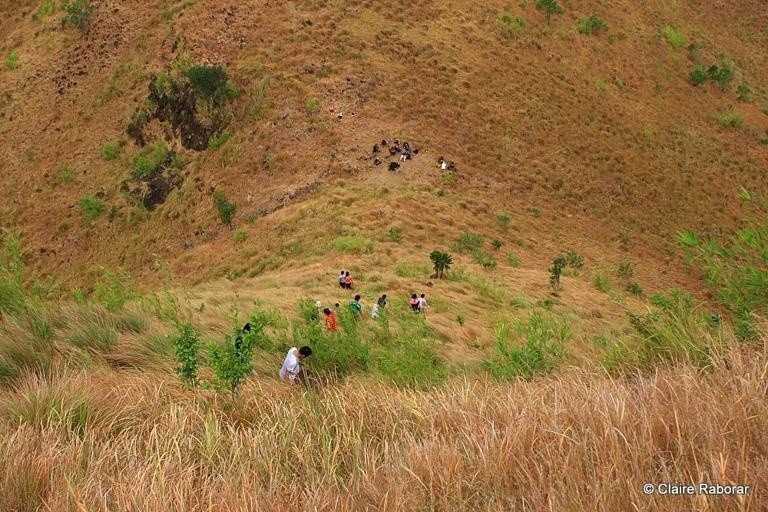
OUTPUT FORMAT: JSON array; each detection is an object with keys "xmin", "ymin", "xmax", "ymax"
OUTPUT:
[
  {"xmin": 344, "ymin": 272, "xmax": 353, "ymax": 289},
  {"xmin": 371, "ymin": 138, "xmax": 419, "ymax": 172},
  {"xmin": 338, "ymin": 270, "xmax": 346, "ymax": 288},
  {"xmin": 233, "ymin": 292, "xmax": 429, "ymax": 375},
  {"xmin": 278, "ymin": 345, "xmax": 312, "ymax": 388},
  {"xmin": 327, "ymin": 105, "xmax": 358, "ymax": 119},
  {"xmin": 436, "ymin": 156, "xmax": 457, "ymax": 172}
]
